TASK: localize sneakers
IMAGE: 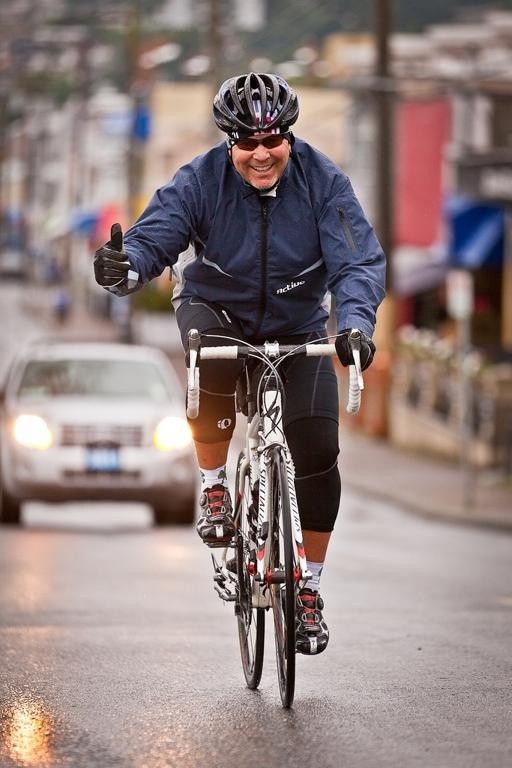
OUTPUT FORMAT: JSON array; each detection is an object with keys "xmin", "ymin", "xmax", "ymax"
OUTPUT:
[
  {"xmin": 295, "ymin": 587, "xmax": 329, "ymax": 655},
  {"xmin": 195, "ymin": 482, "xmax": 235, "ymax": 541}
]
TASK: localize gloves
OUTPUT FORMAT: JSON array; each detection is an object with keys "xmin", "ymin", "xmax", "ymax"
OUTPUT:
[
  {"xmin": 335, "ymin": 327, "xmax": 376, "ymax": 371},
  {"xmin": 93, "ymin": 222, "xmax": 131, "ymax": 292}
]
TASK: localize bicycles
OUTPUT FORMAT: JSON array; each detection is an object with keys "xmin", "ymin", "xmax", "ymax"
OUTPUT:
[{"xmin": 181, "ymin": 322, "xmax": 366, "ymax": 713}]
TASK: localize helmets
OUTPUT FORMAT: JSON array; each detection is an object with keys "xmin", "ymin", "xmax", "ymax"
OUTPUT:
[{"xmin": 212, "ymin": 71, "xmax": 300, "ymax": 146}]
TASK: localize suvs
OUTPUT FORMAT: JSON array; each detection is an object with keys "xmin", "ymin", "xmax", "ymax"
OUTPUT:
[{"xmin": 0, "ymin": 331, "xmax": 201, "ymax": 530}]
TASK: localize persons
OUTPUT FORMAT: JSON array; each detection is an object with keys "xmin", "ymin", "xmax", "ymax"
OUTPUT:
[{"xmin": 94, "ymin": 73, "xmax": 387, "ymax": 654}]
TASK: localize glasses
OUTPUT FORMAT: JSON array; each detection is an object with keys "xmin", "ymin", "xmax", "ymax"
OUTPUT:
[{"xmin": 236, "ymin": 134, "xmax": 285, "ymax": 151}]
